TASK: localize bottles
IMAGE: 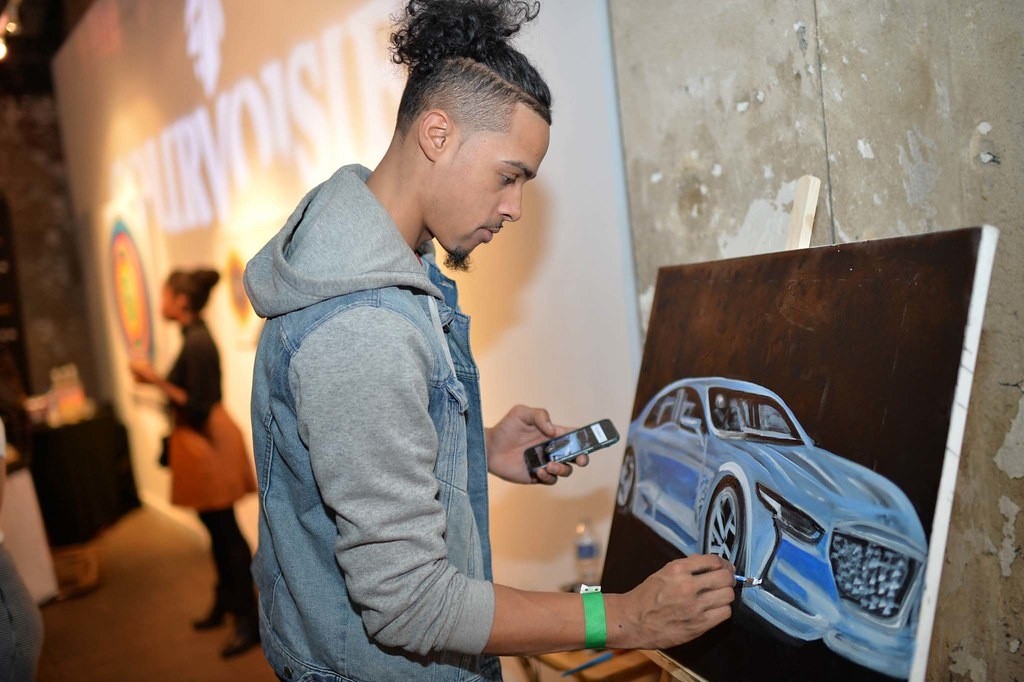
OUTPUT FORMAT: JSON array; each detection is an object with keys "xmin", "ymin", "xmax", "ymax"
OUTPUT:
[{"xmin": 575, "ymin": 515, "xmax": 601, "ymax": 586}]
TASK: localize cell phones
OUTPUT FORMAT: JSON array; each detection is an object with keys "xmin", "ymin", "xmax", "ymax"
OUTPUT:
[{"xmin": 524, "ymin": 419, "xmax": 620, "ymax": 474}]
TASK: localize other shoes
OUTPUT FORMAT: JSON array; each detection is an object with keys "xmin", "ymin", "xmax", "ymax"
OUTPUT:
[
  {"xmin": 220, "ymin": 629, "xmax": 261, "ymax": 658},
  {"xmin": 194, "ymin": 602, "xmax": 233, "ymax": 629}
]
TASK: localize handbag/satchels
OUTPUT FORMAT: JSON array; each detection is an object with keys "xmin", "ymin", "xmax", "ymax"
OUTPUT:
[{"xmin": 167, "ymin": 401, "xmax": 258, "ymax": 510}]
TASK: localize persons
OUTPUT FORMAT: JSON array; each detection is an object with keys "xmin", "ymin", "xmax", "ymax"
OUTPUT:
[
  {"xmin": 244, "ymin": 0, "xmax": 736, "ymax": 682},
  {"xmin": 128, "ymin": 270, "xmax": 257, "ymax": 655}
]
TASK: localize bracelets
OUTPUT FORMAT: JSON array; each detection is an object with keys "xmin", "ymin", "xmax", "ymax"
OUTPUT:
[{"xmin": 578, "ymin": 582, "xmax": 610, "ymax": 650}]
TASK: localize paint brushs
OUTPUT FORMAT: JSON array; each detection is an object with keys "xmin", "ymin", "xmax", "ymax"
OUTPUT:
[{"xmin": 734, "ymin": 574, "xmax": 763, "ymax": 587}]
